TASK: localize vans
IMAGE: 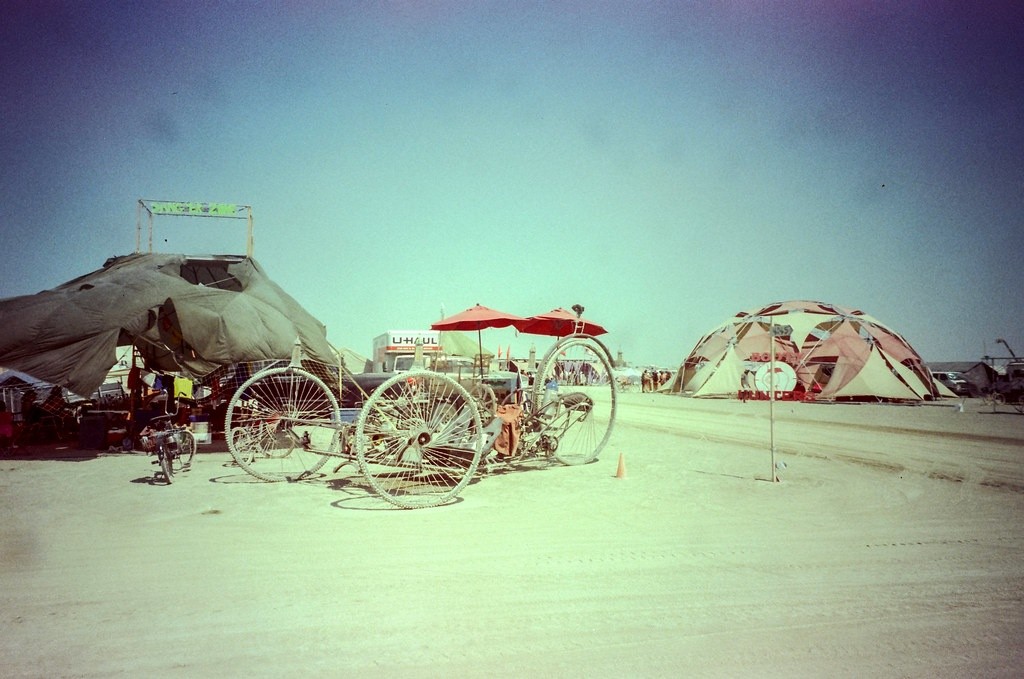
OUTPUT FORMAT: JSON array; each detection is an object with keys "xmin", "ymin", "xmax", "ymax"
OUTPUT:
[{"xmin": 932, "ymin": 371, "xmax": 970, "ymax": 396}]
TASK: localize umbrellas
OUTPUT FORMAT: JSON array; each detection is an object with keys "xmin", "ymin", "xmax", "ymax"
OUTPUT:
[
  {"xmin": 512, "ymin": 305, "xmax": 611, "ymax": 384},
  {"xmin": 428, "ymin": 303, "xmax": 532, "ymax": 384},
  {"xmin": 437, "ymin": 332, "xmax": 496, "ymax": 376}
]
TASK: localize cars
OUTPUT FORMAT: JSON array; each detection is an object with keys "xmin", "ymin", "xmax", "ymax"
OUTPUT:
[{"xmin": 429, "ymin": 357, "xmax": 530, "ymax": 397}]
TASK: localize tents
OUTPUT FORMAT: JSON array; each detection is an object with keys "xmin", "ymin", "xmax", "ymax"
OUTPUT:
[
  {"xmin": 0, "ymin": 251, "xmax": 400, "ymax": 437},
  {"xmin": 0, "ymin": 369, "xmax": 37, "ymax": 414},
  {"xmin": 657, "ymin": 299, "xmax": 959, "ymax": 405},
  {"xmin": 337, "ymin": 347, "xmax": 373, "ymax": 375}
]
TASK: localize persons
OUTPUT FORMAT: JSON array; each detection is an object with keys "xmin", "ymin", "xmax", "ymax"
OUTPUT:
[
  {"xmin": 649, "ymin": 369, "xmax": 659, "ymax": 391},
  {"xmin": 20, "ymin": 390, "xmax": 51, "ymax": 434},
  {"xmin": 0, "ymin": 400, "xmax": 19, "ymax": 452},
  {"xmin": 740, "ymin": 368, "xmax": 751, "ymax": 404},
  {"xmin": 640, "ymin": 369, "xmax": 653, "ymax": 393},
  {"xmin": 664, "ymin": 370, "xmax": 672, "ymax": 382},
  {"xmin": 659, "ymin": 370, "xmax": 666, "ymax": 386}
]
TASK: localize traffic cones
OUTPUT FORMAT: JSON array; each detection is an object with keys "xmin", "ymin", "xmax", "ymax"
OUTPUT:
[{"xmin": 615, "ymin": 452, "xmax": 627, "ymax": 478}]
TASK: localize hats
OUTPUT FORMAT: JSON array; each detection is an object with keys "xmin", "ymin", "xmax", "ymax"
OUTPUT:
[{"xmin": 745, "ymin": 368, "xmax": 750, "ymax": 371}]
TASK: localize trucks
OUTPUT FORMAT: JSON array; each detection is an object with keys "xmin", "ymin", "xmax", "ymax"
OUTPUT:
[{"xmin": 372, "ymin": 329, "xmax": 441, "ymax": 375}]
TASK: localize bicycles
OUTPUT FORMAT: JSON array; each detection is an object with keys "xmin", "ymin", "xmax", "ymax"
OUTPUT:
[
  {"xmin": 983, "ymin": 388, "xmax": 1007, "ymax": 406},
  {"xmin": 148, "ymin": 389, "xmax": 199, "ymax": 484},
  {"xmin": 615, "ymin": 376, "xmax": 640, "ymax": 394},
  {"xmin": 231, "ymin": 401, "xmax": 297, "ymax": 467}
]
